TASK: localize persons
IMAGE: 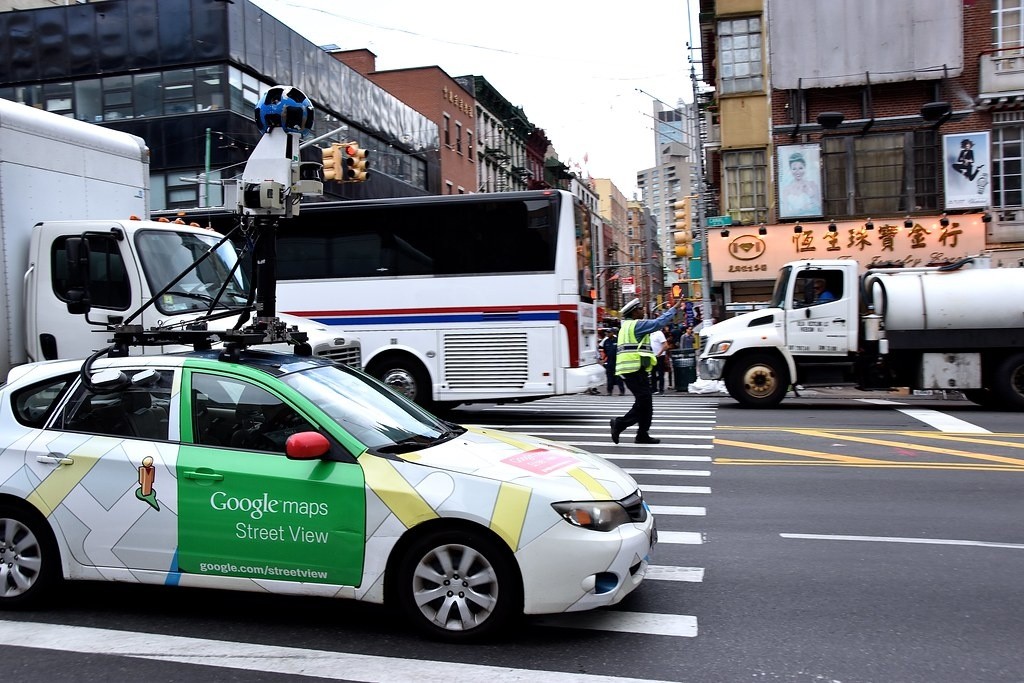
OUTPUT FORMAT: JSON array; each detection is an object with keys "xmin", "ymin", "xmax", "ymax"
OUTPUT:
[
  {"xmin": 812, "ymin": 280, "xmax": 837, "ymax": 307},
  {"xmin": 779, "ymin": 153, "xmax": 820, "ymax": 217},
  {"xmin": 951, "ymin": 139, "xmax": 985, "ymax": 182},
  {"xmin": 588, "ymin": 285, "xmax": 705, "ymax": 447}
]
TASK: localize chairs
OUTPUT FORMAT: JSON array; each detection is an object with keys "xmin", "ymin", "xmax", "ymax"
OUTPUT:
[
  {"xmin": 71, "ymin": 393, "xmax": 167, "ymax": 442},
  {"xmin": 231, "ymin": 384, "xmax": 265, "ymax": 447}
]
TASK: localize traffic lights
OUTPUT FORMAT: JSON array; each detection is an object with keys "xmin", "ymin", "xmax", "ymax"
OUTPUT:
[
  {"xmin": 673, "ymin": 198, "xmax": 694, "ymax": 258},
  {"xmin": 322, "ymin": 145, "xmax": 344, "ymax": 182},
  {"xmin": 356, "ymin": 148, "xmax": 369, "ymax": 182},
  {"xmin": 580, "ymin": 209, "xmax": 593, "ymax": 265},
  {"xmin": 346, "ymin": 141, "xmax": 360, "ymax": 182}
]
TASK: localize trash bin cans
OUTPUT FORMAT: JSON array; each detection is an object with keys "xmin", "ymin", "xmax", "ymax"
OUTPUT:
[{"xmin": 668, "ymin": 348, "xmax": 696, "ymax": 392}]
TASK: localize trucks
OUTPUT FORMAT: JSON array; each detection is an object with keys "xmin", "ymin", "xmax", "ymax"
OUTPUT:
[
  {"xmin": 697, "ymin": 256, "xmax": 1024, "ymax": 411},
  {"xmin": 2, "ymin": 97, "xmax": 362, "ymax": 437}
]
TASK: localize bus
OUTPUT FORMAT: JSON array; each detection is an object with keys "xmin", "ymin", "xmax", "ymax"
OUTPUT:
[{"xmin": 142, "ymin": 188, "xmax": 607, "ymax": 418}]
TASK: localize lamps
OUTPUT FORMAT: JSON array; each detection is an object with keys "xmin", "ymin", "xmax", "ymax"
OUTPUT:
[
  {"xmin": 904, "ymin": 220, "xmax": 913, "ymax": 229},
  {"xmin": 982, "ymin": 215, "xmax": 993, "ymax": 223},
  {"xmin": 940, "ymin": 218, "xmax": 949, "ymax": 226},
  {"xmin": 865, "ymin": 222, "xmax": 874, "ymax": 231},
  {"xmin": 67, "ymin": 277, "xmax": 103, "ymax": 308},
  {"xmin": 828, "ymin": 224, "xmax": 837, "ymax": 232},
  {"xmin": 720, "ymin": 229, "xmax": 729, "ymax": 238},
  {"xmin": 794, "ymin": 226, "xmax": 802, "ymax": 233},
  {"xmin": 759, "ymin": 227, "xmax": 767, "ymax": 235}
]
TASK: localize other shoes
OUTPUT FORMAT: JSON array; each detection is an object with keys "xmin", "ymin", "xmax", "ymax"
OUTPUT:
[
  {"xmin": 635, "ymin": 435, "xmax": 660, "ymax": 444},
  {"xmin": 605, "ymin": 392, "xmax": 611, "ymax": 396},
  {"xmin": 668, "ymin": 386, "xmax": 674, "ymax": 389},
  {"xmin": 618, "ymin": 393, "xmax": 624, "ymax": 396},
  {"xmin": 611, "ymin": 418, "xmax": 624, "ymax": 444},
  {"xmin": 652, "ymin": 391, "xmax": 659, "ymax": 394}
]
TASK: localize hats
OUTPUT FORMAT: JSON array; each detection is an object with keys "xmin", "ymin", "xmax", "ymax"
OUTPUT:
[
  {"xmin": 606, "ymin": 328, "xmax": 614, "ymax": 332},
  {"xmin": 619, "ymin": 298, "xmax": 642, "ymax": 316}
]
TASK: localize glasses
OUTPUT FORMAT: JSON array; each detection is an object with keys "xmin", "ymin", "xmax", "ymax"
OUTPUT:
[{"xmin": 635, "ymin": 307, "xmax": 643, "ymax": 310}]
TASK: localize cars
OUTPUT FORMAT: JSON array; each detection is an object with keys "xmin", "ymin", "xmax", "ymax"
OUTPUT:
[{"xmin": 1, "ymin": 85, "xmax": 657, "ymax": 646}]
